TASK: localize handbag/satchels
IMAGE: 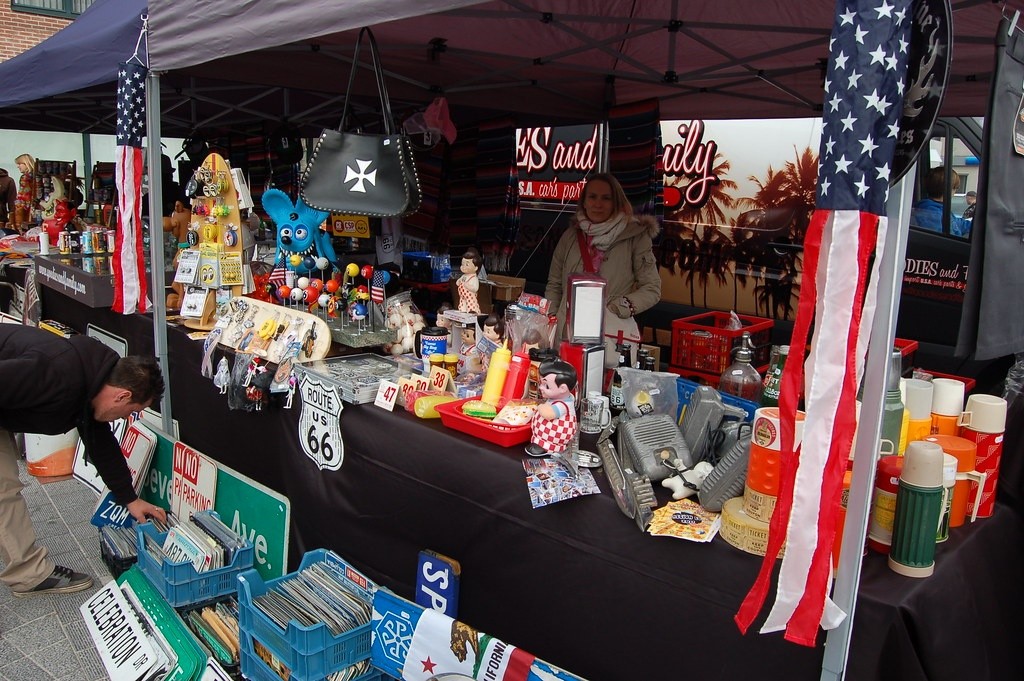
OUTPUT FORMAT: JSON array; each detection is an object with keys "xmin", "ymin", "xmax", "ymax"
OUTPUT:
[{"xmin": 300, "ymin": 127, "xmax": 422, "ymax": 218}]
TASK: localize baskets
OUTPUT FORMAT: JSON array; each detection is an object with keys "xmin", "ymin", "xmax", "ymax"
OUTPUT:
[
  {"xmin": 237, "ymin": 548, "xmax": 384, "ymax": 681},
  {"xmin": 134, "ymin": 510, "xmax": 255, "ymax": 608}
]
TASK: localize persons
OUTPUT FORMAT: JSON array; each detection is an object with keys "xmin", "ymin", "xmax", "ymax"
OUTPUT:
[
  {"xmin": 455, "ymin": 247, "xmax": 481, "ymax": 313},
  {"xmin": 456, "ymin": 325, "xmax": 482, "ymax": 373},
  {"xmin": 544, "ymin": 172, "xmax": 662, "ymax": 368},
  {"xmin": 482, "ymin": 312, "xmax": 503, "ymax": 345},
  {"xmin": 962, "ymin": 190, "xmax": 977, "ymax": 219},
  {"xmin": 142, "ymin": 153, "xmax": 191, "ymax": 217},
  {"xmin": 531, "ymin": 353, "xmax": 577, "ymax": 453},
  {"xmin": 914, "ymin": 166, "xmax": 972, "ymax": 236},
  {"xmin": 436, "ymin": 301, "xmax": 454, "ymax": 346},
  {"xmin": 0, "ymin": 323, "xmax": 166, "ymax": 596},
  {"xmin": 15, "ymin": 153, "xmax": 35, "ymax": 206}
]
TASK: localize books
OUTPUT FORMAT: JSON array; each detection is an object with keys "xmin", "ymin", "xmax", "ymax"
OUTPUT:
[
  {"xmin": 324, "ymin": 660, "xmax": 371, "ymax": 681},
  {"xmin": 101, "ymin": 509, "xmax": 180, "ymax": 557},
  {"xmin": 250, "ymin": 562, "xmax": 373, "ymax": 636},
  {"xmin": 190, "ymin": 595, "xmax": 239, "ymax": 663},
  {"xmin": 162, "ymin": 510, "xmax": 247, "ymax": 574}
]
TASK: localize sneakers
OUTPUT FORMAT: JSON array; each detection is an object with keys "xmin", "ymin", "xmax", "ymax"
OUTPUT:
[{"xmin": 12, "ymin": 566, "xmax": 92, "ymax": 596}]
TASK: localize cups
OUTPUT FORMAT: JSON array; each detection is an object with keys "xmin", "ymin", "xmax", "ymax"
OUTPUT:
[
  {"xmin": 414, "ymin": 326, "xmax": 451, "ymax": 359},
  {"xmin": 580, "ymin": 398, "xmax": 612, "ymax": 435},
  {"xmin": 528, "ymin": 347, "xmax": 559, "ymax": 400}
]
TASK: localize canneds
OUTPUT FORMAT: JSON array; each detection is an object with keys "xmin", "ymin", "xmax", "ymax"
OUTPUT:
[
  {"xmin": 61, "ymin": 256, "xmax": 115, "ymax": 275},
  {"xmin": 38, "ymin": 231, "xmax": 50, "ymax": 255},
  {"xmin": 93, "ymin": 176, "xmax": 112, "ymax": 224},
  {"xmin": 59, "ymin": 230, "xmax": 115, "ymax": 255},
  {"xmin": 35, "ymin": 161, "xmax": 65, "ymax": 223}
]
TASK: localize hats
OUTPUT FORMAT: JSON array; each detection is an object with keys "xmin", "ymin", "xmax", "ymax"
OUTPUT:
[
  {"xmin": 185, "ymin": 136, "xmax": 209, "ymax": 165},
  {"xmin": 161, "ymin": 154, "xmax": 176, "ymax": 174},
  {"xmin": 967, "ymin": 191, "xmax": 977, "ymax": 197},
  {"xmin": 271, "ymin": 126, "xmax": 303, "ymax": 163},
  {"xmin": 425, "ymin": 97, "xmax": 457, "ymax": 144}
]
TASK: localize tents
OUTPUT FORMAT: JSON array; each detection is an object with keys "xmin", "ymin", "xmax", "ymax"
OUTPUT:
[{"xmin": 0, "ymin": 1, "xmax": 1024, "ymax": 430}]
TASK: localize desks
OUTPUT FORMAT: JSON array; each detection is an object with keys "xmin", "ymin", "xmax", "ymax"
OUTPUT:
[{"xmin": 0, "ymin": 234, "xmax": 1024, "ymax": 681}]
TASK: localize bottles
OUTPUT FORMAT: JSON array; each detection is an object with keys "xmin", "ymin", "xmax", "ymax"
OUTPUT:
[
  {"xmin": 610, "ymin": 344, "xmax": 655, "ymax": 412},
  {"xmin": 497, "ymin": 341, "xmax": 530, "ymax": 409},
  {"xmin": 720, "ymin": 330, "xmax": 1007, "ymax": 579},
  {"xmin": 480, "ymin": 339, "xmax": 512, "ymax": 407}
]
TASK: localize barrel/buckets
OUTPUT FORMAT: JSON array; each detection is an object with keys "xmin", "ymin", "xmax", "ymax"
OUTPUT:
[{"xmin": 24, "ymin": 427, "xmax": 79, "ymax": 477}]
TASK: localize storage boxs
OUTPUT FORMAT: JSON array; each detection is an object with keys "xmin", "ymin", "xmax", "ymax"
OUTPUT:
[
  {"xmin": 893, "ymin": 334, "xmax": 975, "ymax": 406},
  {"xmin": 401, "ymin": 249, "xmax": 451, "ymax": 283},
  {"xmin": 670, "ymin": 312, "xmax": 773, "ymax": 397},
  {"xmin": 486, "ymin": 272, "xmax": 528, "ymax": 300},
  {"xmin": 98, "ymin": 505, "xmax": 395, "ymax": 681},
  {"xmin": 34, "ymin": 251, "xmax": 176, "ymax": 308}
]
